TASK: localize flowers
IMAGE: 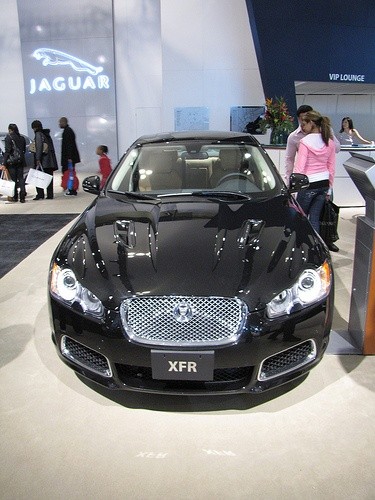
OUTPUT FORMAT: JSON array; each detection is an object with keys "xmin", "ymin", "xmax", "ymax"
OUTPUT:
[{"xmin": 260, "ymin": 97, "xmax": 293, "ymax": 136}]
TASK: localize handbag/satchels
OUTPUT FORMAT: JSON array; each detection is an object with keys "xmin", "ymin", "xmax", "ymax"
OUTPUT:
[
  {"xmin": 25, "ymin": 163, "xmax": 53, "ymax": 189},
  {"xmin": 0, "ymin": 169, "xmax": 15, "ymax": 197},
  {"xmin": 320, "ymin": 198, "xmax": 339, "ymax": 243},
  {"xmin": 60, "ymin": 161, "xmax": 79, "ymax": 190},
  {"xmin": 29, "ymin": 141, "xmax": 48, "ymax": 153},
  {"xmin": 5, "ymin": 134, "xmax": 22, "ymax": 165}
]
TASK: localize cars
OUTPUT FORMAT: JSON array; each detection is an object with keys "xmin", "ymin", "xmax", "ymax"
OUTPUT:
[{"xmin": 47, "ymin": 128, "xmax": 337, "ymax": 398}]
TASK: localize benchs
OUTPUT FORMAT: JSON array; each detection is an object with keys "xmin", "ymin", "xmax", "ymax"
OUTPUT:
[{"xmin": 179, "ymin": 158, "xmax": 219, "ymax": 187}]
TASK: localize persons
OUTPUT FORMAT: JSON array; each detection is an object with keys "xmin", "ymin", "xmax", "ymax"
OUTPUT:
[
  {"xmin": 0, "ymin": 147, "xmax": 7, "ymax": 196},
  {"xmin": 284, "ymin": 105, "xmax": 340, "ymax": 252},
  {"xmin": 293, "ymin": 111, "xmax": 335, "ymax": 242},
  {"xmin": 3, "ymin": 123, "xmax": 26, "ymax": 203},
  {"xmin": 59, "ymin": 117, "xmax": 81, "ymax": 196},
  {"xmin": 31, "ymin": 120, "xmax": 53, "ymax": 200},
  {"xmin": 96, "ymin": 145, "xmax": 112, "ymax": 193},
  {"xmin": 338, "ymin": 117, "xmax": 374, "ymax": 146}
]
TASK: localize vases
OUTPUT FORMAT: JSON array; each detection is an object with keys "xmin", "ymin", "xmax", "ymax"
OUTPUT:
[{"xmin": 270, "ymin": 129, "xmax": 287, "ymax": 145}]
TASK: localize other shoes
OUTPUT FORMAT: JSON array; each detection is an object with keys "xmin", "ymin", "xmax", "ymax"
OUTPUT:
[
  {"xmin": 64, "ymin": 191, "xmax": 77, "ymax": 197},
  {"xmin": 33, "ymin": 194, "xmax": 44, "ymax": 200},
  {"xmin": 46, "ymin": 196, "xmax": 53, "ymax": 199},
  {"xmin": 19, "ymin": 198, "xmax": 26, "ymax": 203},
  {"xmin": 8, "ymin": 197, "xmax": 18, "ymax": 201},
  {"xmin": 326, "ymin": 243, "xmax": 339, "ymax": 252}
]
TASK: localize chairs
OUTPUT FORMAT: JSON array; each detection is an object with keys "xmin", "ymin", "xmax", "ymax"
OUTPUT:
[
  {"xmin": 208, "ymin": 149, "xmax": 253, "ymax": 189},
  {"xmin": 149, "ymin": 151, "xmax": 182, "ymax": 191}
]
